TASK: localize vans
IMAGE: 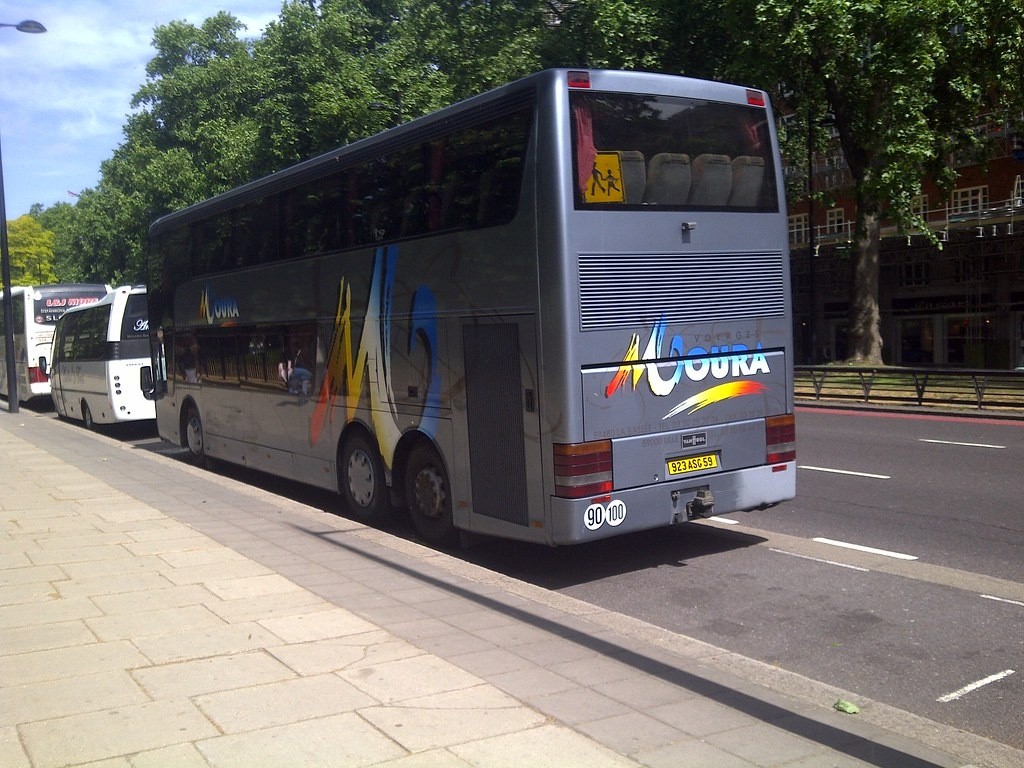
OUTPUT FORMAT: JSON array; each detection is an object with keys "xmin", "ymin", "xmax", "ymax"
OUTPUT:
[{"xmin": 38, "ymin": 284, "xmax": 167, "ymax": 430}]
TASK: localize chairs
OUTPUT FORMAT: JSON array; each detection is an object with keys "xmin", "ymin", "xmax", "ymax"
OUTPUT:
[
  {"xmin": 727, "ymin": 156, "xmax": 764, "ymax": 204},
  {"xmin": 318, "ymin": 174, "xmax": 504, "ymax": 251},
  {"xmin": 616, "ymin": 149, "xmax": 646, "ymax": 203},
  {"xmin": 688, "ymin": 154, "xmax": 732, "ymax": 205},
  {"xmin": 641, "ymin": 153, "xmax": 691, "ymax": 204}
]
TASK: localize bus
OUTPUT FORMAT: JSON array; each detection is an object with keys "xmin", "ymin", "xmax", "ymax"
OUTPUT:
[
  {"xmin": 0, "ymin": 283, "xmax": 113, "ymax": 407},
  {"xmin": 141, "ymin": 67, "xmax": 800, "ymax": 551}
]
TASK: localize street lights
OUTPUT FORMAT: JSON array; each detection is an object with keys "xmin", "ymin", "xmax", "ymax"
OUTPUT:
[{"xmin": 0, "ymin": 20, "xmax": 47, "ymax": 412}]
